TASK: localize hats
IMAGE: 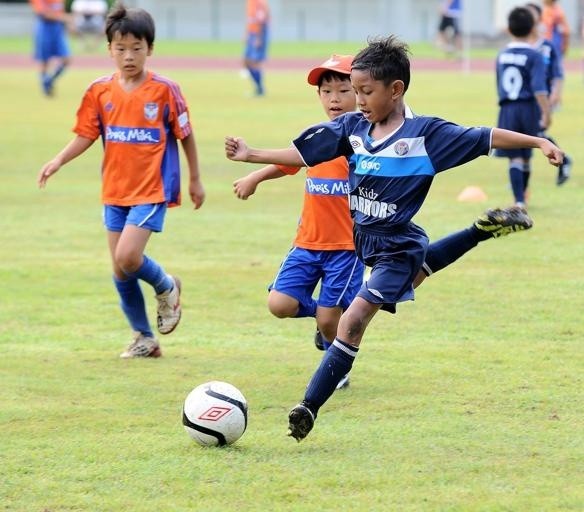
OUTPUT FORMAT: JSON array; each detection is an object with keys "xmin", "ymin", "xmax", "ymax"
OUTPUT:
[{"xmin": 306, "ymin": 53, "xmax": 355, "ymax": 87}]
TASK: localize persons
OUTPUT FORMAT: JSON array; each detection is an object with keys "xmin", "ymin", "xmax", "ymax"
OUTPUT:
[
  {"xmin": 243, "ymin": 0, "xmax": 270, "ymax": 96},
  {"xmin": 38, "ymin": 4, "xmax": 205, "ymax": 359},
  {"xmin": 438, "ymin": 1, "xmax": 465, "ymax": 63},
  {"xmin": 488, "ymin": 0, "xmax": 570, "ymax": 210},
  {"xmin": 233, "ymin": 53, "xmax": 362, "ymax": 391},
  {"xmin": 223, "ymin": 35, "xmax": 564, "ymax": 443},
  {"xmin": 31, "ymin": 0, "xmax": 78, "ymax": 99}
]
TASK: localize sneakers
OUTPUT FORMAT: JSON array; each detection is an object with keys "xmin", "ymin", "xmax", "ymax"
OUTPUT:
[
  {"xmin": 513, "ymin": 156, "xmax": 573, "ymax": 207},
  {"xmin": 314, "ymin": 323, "xmax": 325, "ymax": 351},
  {"xmin": 473, "ymin": 206, "xmax": 532, "ymax": 240},
  {"xmin": 285, "ymin": 406, "xmax": 316, "ymax": 444},
  {"xmin": 119, "ymin": 331, "xmax": 163, "ymax": 360},
  {"xmin": 253, "ymin": 89, "xmax": 265, "ymax": 96},
  {"xmin": 40, "ymin": 79, "xmax": 53, "ymax": 96},
  {"xmin": 152, "ymin": 273, "xmax": 183, "ymax": 336},
  {"xmin": 334, "ymin": 373, "xmax": 350, "ymax": 391}
]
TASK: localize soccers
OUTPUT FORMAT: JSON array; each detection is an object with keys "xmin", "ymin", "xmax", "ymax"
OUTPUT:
[{"xmin": 183, "ymin": 381, "xmax": 247, "ymax": 446}]
